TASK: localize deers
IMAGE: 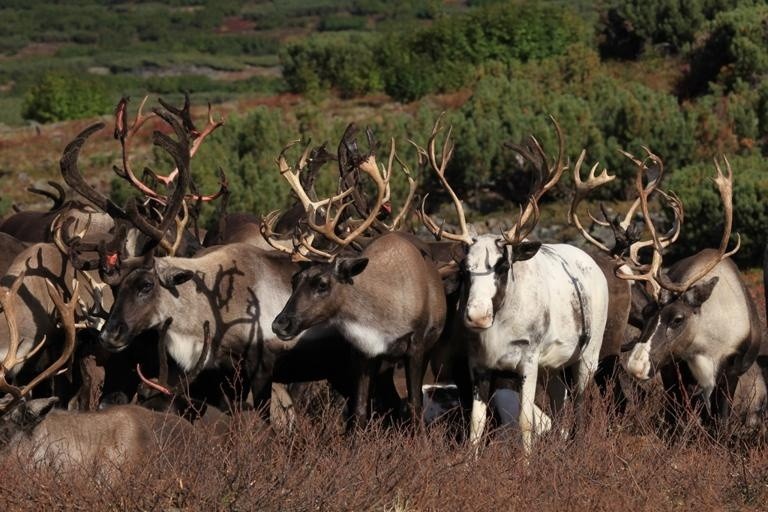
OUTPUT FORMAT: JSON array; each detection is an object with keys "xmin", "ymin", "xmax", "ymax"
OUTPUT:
[{"xmin": 0, "ymin": 88, "xmax": 768, "ymax": 500}]
